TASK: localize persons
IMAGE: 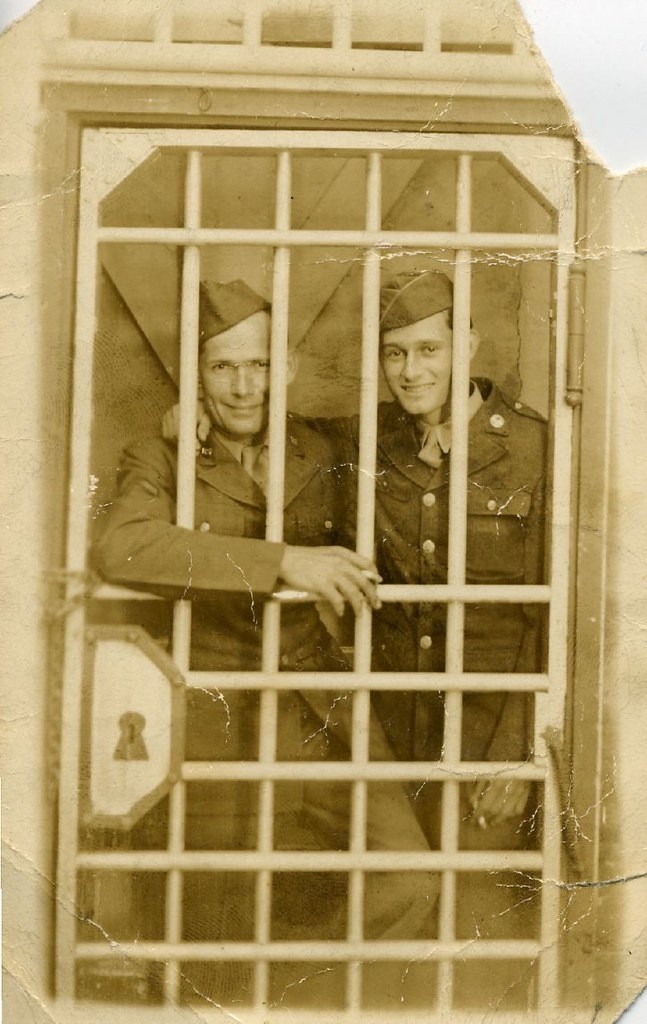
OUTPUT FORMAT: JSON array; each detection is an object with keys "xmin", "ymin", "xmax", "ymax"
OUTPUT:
[
  {"xmin": 161, "ymin": 271, "xmax": 556, "ymax": 1007},
  {"xmin": 91, "ymin": 281, "xmax": 442, "ymax": 1010}
]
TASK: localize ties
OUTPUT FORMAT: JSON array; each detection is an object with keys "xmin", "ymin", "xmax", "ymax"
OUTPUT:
[
  {"xmin": 417, "ymin": 428, "xmax": 444, "ymax": 469},
  {"xmin": 241, "ymin": 445, "xmax": 265, "ymax": 477}
]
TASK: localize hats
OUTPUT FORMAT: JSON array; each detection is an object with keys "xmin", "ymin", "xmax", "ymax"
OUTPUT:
[
  {"xmin": 197, "ymin": 278, "xmax": 272, "ymax": 345},
  {"xmin": 379, "ymin": 269, "xmax": 453, "ymax": 332}
]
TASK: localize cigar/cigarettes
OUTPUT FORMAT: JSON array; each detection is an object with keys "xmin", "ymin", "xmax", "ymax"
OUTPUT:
[{"xmin": 362, "ymin": 570, "xmax": 383, "ymax": 582}]
